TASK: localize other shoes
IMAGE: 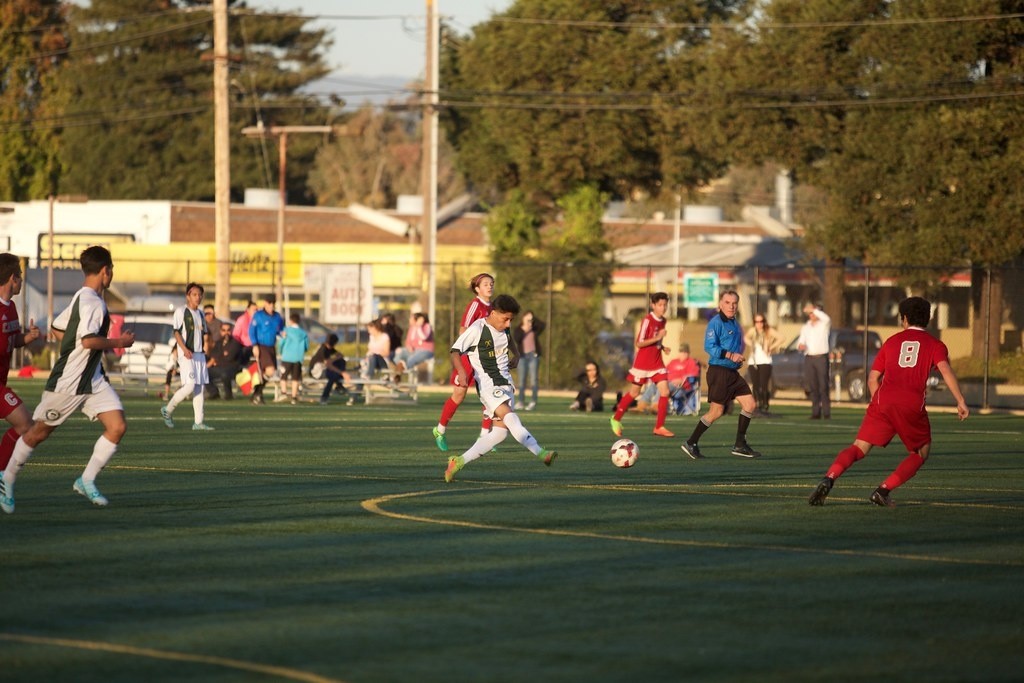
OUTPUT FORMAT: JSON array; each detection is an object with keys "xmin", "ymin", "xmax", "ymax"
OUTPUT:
[
  {"xmin": 525, "ymin": 403, "xmax": 536, "ymax": 411},
  {"xmin": 514, "ymin": 403, "xmax": 524, "ymax": 410},
  {"xmin": 570, "ymin": 406, "xmax": 578, "ymax": 410},
  {"xmin": 586, "ymin": 398, "xmax": 593, "ymax": 413}
]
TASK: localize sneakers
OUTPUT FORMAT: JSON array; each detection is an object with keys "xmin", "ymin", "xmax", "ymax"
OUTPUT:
[
  {"xmin": 731, "ymin": 443, "xmax": 761, "ymax": 458},
  {"xmin": 654, "ymin": 426, "xmax": 675, "ymax": 437},
  {"xmin": 544, "ymin": 451, "xmax": 557, "ymax": 466},
  {"xmin": 0, "ymin": 471, "xmax": 15, "ymax": 513},
  {"xmin": 161, "ymin": 407, "xmax": 174, "ymax": 428},
  {"xmin": 192, "ymin": 422, "xmax": 214, "ymax": 431},
  {"xmin": 809, "ymin": 477, "xmax": 832, "ymax": 506},
  {"xmin": 611, "ymin": 415, "xmax": 624, "ymax": 436},
  {"xmin": 433, "ymin": 427, "xmax": 448, "ymax": 453},
  {"xmin": 681, "ymin": 441, "xmax": 705, "ymax": 460},
  {"xmin": 444, "ymin": 456, "xmax": 459, "ymax": 482},
  {"xmin": 870, "ymin": 490, "xmax": 896, "ymax": 507},
  {"xmin": 74, "ymin": 477, "xmax": 108, "ymax": 506}
]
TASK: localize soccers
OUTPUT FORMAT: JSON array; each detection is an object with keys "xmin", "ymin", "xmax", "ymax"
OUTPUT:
[{"xmin": 610, "ymin": 438, "xmax": 639, "ymax": 468}]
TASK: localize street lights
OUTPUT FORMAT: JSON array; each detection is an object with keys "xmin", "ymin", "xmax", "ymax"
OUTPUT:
[
  {"xmin": 45, "ymin": 190, "xmax": 90, "ymax": 368},
  {"xmin": 670, "ymin": 179, "xmax": 715, "ymax": 320}
]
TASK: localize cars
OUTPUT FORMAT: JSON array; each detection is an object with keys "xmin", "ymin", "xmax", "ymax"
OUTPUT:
[
  {"xmin": 105, "ymin": 316, "xmax": 181, "ymax": 384},
  {"xmin": 574, "ymin": 330, "xmax": 636, "ymax": 381}
]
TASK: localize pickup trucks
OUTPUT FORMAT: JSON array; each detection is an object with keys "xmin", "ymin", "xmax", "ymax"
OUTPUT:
[{"xmin": 744, "ymin": 327, "xmax": 946, "ymax": 404}]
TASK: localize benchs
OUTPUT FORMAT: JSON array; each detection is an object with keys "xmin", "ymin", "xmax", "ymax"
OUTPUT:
[
  {"xmin": 268, "ymin": 357, "xmax": 433, "ymax": 406},
  {"xmin": 105, "ymin": 354, "xmax": 181, "ymax": 393}
]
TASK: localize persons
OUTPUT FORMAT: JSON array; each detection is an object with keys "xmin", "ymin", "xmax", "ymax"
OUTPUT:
[
  {"xmin": 163, "ymin": 295, "xmax": 310, "ymax": 406},
  {"xmin": 0, "ymin": 246, "xmax": 134, "ymax": 513},
  {"xmin": 680, "ymin": 291, "xmax": 761, "ymax": 459},
  {"xmin": 569, "ymin": 362, "xmax": 606, "ymax": 411},
  {"xmin": 808, "ymin": 297, "xmax": 969, "ymax": 507},
  {"xmin": 611, "ymin": 344, "xmax": 701, "ymax": 416},
  {"xmin": 346, "ymin": 313, "xmax": 436, "ymax": 406},
  {"xmin": 309, "ymin": 334, "xmax": 351, "ymax": 406},
  {"xmin": 796, "ymin": 303, "xmax": 832, "ymax": 420},
  {"xmin": 514, "ymin": 311, "xmax": 546, "ymax": 411},
  {"xmin": 433, "ymin": 273, "xmax": 496, "ymax": 452},
  {"xmin": 743, "ymin": 314, "xmax": 785, "ymax": 419},
  {"xmin": 609, "ymin": 292, "xmax": 675, "ymax": 437},
  {"xmin": 0, "ymin": 253, "xmax": 39, "ymax": 471},
  {"xmin": 160, "ymin": 282, "xmax": 215, "ymax": 431},
  {"xmin": 443, "ymin": 295, "xmax": 558, "ymax": 483}
]
